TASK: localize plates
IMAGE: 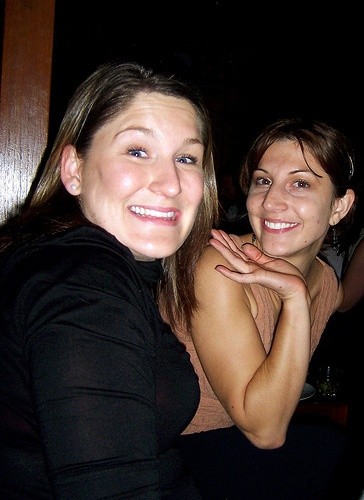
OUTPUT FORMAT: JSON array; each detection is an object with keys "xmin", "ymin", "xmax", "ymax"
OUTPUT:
[{"xmin": 299, "ymin": 383, "xmax": 314, "ymax": 401}]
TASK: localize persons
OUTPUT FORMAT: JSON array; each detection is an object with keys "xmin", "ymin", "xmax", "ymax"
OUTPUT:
[
  {"xmin": 1, "ymin": 62, "xmax": 220, "ymax": 500},
  {"xmin": 153, "ymin": 119, "xmax": 362, "ymax": 500},
  {"xmin": 308, "ymin": 181, "xmax": 362, "ymax": 403}
]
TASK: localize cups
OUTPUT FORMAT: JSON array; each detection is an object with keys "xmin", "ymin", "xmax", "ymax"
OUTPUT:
[{"xmin": 315, "ymin": 363, "xmax": 344, "ymax": 399}]
